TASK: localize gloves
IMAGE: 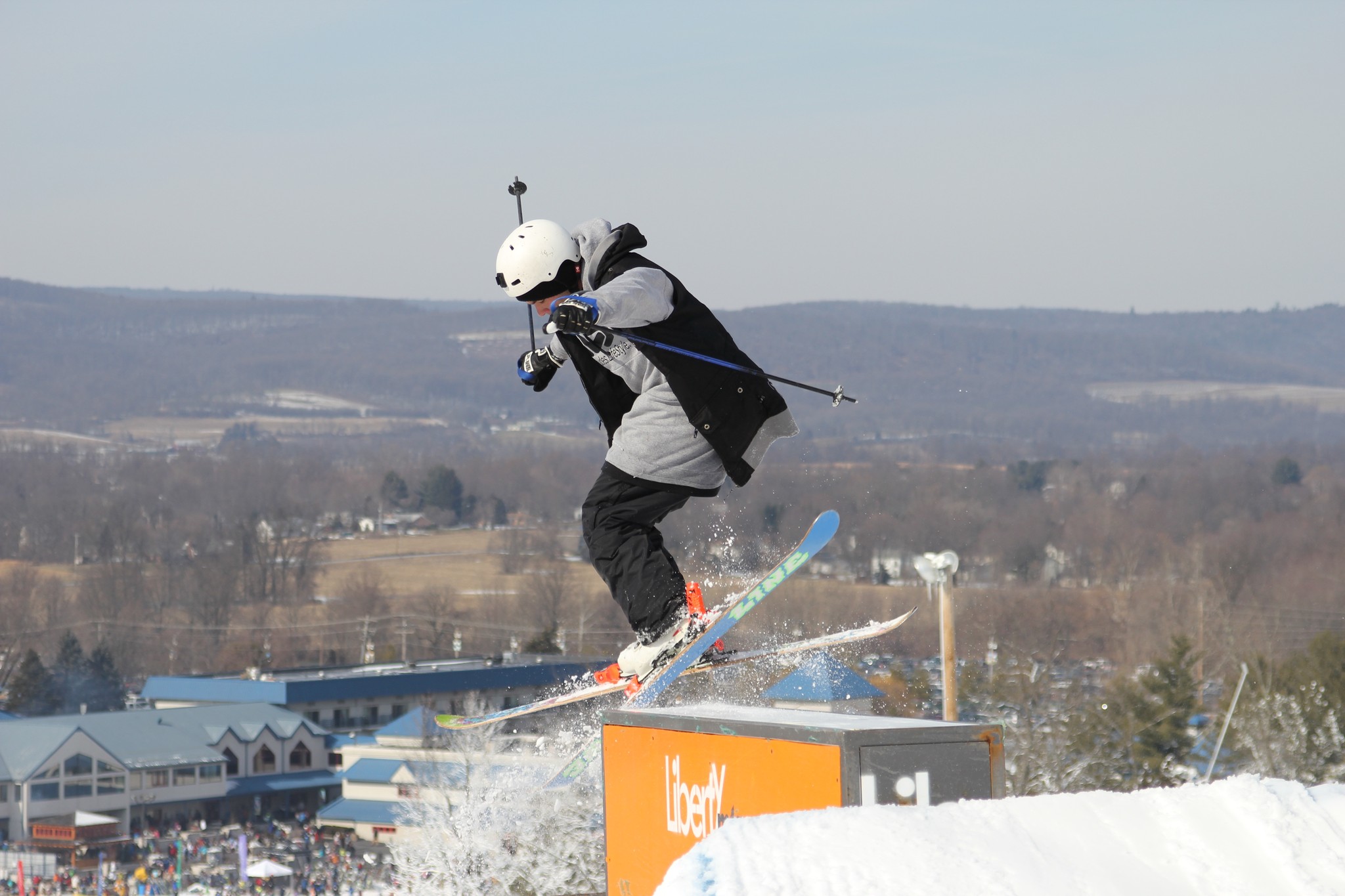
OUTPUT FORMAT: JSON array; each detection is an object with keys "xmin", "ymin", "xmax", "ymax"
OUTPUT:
[
  {"xmin": 542, "ymin": 300, "xmax": 595, "ymax": 334},
  {"xmin": 518, "ymin": 345, "xmax": 563, "ymax": 392}
]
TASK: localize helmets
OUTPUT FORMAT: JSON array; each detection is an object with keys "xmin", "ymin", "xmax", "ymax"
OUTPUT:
[{"xmin": 496, "ymin": 219, "xmax": 581, "ymax": 298}]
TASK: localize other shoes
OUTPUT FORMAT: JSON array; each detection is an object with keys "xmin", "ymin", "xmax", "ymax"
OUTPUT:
[{"xmin": 617, "ymin": 618, "xmax": 689, "ymax": 675}]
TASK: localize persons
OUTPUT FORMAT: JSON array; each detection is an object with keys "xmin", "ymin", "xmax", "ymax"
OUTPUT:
[
  {"xmin": 0, "ymin": 810, "xmax": 398, "ymax": 896},
  {"xmin": 495, "ymin": 219, "xmax": 798, "ymax": 693}
]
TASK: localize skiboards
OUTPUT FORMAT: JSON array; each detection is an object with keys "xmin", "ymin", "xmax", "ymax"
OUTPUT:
[{"xmin": 436, "ymin": 508, "xmax": 916, "ymax": 793}]
